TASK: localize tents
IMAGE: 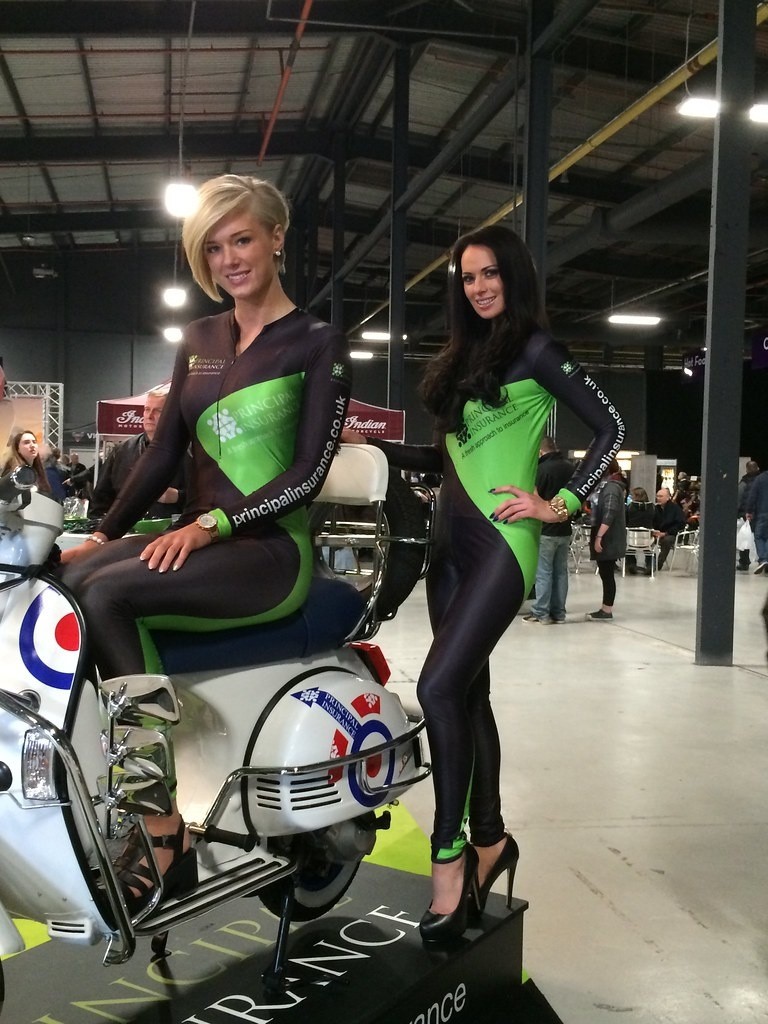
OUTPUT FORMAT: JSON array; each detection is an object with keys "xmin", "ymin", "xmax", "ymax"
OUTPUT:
[{"xmin": 93, "ymin": 374, "xmax": 407, "ymax": 498}]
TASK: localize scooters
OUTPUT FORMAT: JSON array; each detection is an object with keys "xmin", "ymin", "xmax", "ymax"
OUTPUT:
[{"xmin": 0, "ymin": 440, "xmax": 436, "ymax": 1010}]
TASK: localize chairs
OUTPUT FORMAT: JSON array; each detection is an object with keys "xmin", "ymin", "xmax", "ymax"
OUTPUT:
[{"xmin": 568, "ymin": 521, "xmax": 701, "ymax": 581}]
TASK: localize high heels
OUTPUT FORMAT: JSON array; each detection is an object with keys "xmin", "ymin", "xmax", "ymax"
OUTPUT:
[
  {"xmin": 418, "ymin": 842, "xmax": 481, "ymax": 946},
  {"xmin": 97, "ymin": 813, "xmax": 198, "ymax": 917},
  {"xmin": 478, "ymin": 833, "xmax": 519, "ymax": 911}
]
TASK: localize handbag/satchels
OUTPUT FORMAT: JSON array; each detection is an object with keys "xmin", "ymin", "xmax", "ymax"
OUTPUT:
[{"xmin": 736, "ymin": 517, "xmax": 755, "ymax": 551}]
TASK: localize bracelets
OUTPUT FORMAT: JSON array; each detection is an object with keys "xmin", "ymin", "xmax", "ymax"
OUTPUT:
[
  {"xmin": 595, "ymin": 536, "xmax": 602, "ymax": 538},
  {"xmin": 84, "ymin": 536, "xmax": 105, "ymax": 546}
]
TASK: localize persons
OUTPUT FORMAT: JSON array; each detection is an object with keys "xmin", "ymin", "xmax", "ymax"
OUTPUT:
[
  {"xmin": 623, "ymin": 460, "xmax": 701, "ymax": 579},
  {"xmin": 584, "ymin": 456, "xmax": 625, "ymax": 621},
  {"xmin": 0, "ymin": 431, "xmax": 54, "ymax": 502},
  {"xmin": 736, "ymin": 461, "xmax": 768, "ymax": 575},
  {"xmin": 84, "ymin": 380, "xmax": 192, "ymax": 522},
  {"xmin": 40, "ymin": 440, "xmax": 117, "ymax": 510},
  {"xmin": 521, "ymin": 435, "xmax": 579, "ymax": 625},
  {"xmin": 338, "ymin": 225, "xmax": 625, "ymax": 947},
  {"xmin": 55, "ymin": 172, "xmax": 355, "ymax": 934}
]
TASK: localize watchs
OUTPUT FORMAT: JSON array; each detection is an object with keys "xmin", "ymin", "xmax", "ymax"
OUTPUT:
[
  {"xmin": 195, "ymin": 513, "xmax": 220, "ymax": 543},
  {"xmin": 548, "ymin": 496, "xmax": 569, "ymax": 522}
]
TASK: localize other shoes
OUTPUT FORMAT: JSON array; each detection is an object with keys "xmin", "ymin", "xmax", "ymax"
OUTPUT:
[
  {"xmin": 753, "ymin": 561, "xmax": 768, "ymax": 574},
  {"xmin": 521, "ymin": 612, "xmax": 566, "ymax": 624},
  {"xmin": 587, "ymin": 610, "xmax": 614, "ymax": 620}
]
